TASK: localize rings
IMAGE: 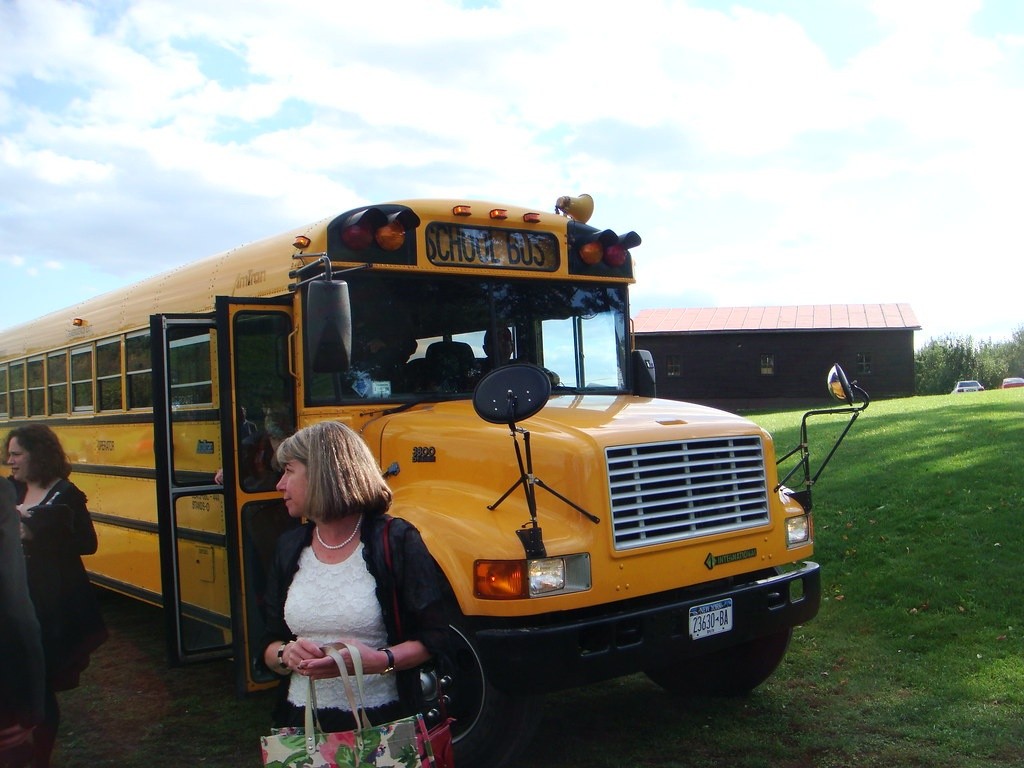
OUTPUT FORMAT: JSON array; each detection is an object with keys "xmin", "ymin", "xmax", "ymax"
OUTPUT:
[{"xmin": 297, "ymin": 664, "xmax": 302, "ymax": 669}]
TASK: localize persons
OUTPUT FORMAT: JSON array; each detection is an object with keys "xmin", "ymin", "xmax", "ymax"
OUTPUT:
[
  {"xmin": 0, "ymin": 478, "xmax": 60, "ymax": 768},
  {"xmin": 3, "ymin": 424, "xmax": 107, "ymax": 691},
  {"xmin": 252, "ymin": 422, "xmax": 449, "ymax": 733},
  {"xmin": 214, "ymin": 400, "xmax": 293, "ymax": 535},
  {"xmin": 472, "ymin": 325, "xmax": 560, "ymax": 387}
]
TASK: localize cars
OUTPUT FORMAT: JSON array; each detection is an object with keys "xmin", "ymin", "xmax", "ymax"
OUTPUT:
[
  {"xmin": 951, "ymin": 381, "xmax": 986, "ymax": 394},
  {"xmin": 1001, "ymin": 377, "xmax": 1024, "ymax": 389}
]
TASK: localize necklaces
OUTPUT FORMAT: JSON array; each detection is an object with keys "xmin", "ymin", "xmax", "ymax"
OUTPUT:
[{"xmin": 315, "ymin": 515, "xmax": 362, "ymax": 548}]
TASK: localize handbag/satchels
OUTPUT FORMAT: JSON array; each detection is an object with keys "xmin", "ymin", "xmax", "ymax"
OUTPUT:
[
  {"xmin": 417, "ymin": 717, "xmax": 456, "ymax": 768},
  {"xmin": 260, "ymin": 642, "xmax": 436, "ymax": 768}
]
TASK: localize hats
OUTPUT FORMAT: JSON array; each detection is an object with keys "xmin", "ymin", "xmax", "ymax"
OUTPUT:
[{"xmin": 484, "ymin": 325, "xmax": 511, "ymax": 345}]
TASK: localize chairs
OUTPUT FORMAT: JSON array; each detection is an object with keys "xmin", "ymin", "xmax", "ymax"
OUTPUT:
[{"xmin": 426, "ymin": 340, "xmax": 481, "ymax": 393}]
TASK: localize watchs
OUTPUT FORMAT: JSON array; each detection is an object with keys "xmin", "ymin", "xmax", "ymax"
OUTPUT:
[{"xmin": 377, "ymin": 647, "xmax": 394, "ymax": 676}]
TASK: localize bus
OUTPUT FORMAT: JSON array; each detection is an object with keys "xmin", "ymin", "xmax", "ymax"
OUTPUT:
[{"xmin": 0, "ymin": 194, "xmax": 873, "ymax": 768}]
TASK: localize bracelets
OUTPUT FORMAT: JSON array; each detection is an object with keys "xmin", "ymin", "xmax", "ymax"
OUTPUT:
[{"xmin": 278, "ymin": 641, "xmax": 293, "ymax": 670}]
